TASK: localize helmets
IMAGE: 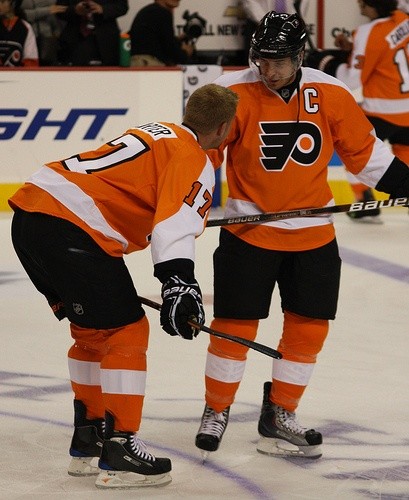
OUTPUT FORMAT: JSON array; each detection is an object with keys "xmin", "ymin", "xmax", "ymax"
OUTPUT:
[{"xmin": 251, "ymin": 10, "xmax": 309, "ymax": 60}]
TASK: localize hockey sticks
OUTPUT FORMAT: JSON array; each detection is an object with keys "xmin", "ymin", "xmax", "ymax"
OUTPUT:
[
  {"xmin": 138, "ymin": 295, "xmax": 284, "ymax": 360},
  {"xmin": 292, "ymin": 0, "xmax": 315, "ymax": 49},
  {"xmin": 206, "ymin": 196, "xmax": 409, "ymax": 228}
]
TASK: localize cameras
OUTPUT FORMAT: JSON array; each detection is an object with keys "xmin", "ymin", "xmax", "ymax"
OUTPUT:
[{"xmin": 181, "ymin": 10, "xmax": 207, "ymax": 36}]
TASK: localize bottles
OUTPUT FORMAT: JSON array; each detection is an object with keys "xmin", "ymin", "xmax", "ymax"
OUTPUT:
[{"xmin": 117, "ymin": 32, "xmax": 131, "ymax": 67}]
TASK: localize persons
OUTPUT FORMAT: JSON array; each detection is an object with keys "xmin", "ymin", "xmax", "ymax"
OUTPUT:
[
  {"xmin": 19, "ymin": 0, "xmax": 68, "ymax": 67},
  {"xmin": 56, "ymin": 0, "xmax": 129, "ymax": 67},
  {"xmin": 0, "ymin": 0, "xmax": 39, "ymax": 67},
  {"xmin": 236, "ymin": 1, "xmax": 259, "ymax": 66},
  {"xmin": 195, "ymin": 11, "xmax": 409, "ymax": 464},
  {"xmin": 306, "ymin": 0, "xmax": 409, "ymax": 223},
  {"xmin": 8, "ymin": 84, "xmax": 240, "ymax": 490},
  {"xmin": 131, "ymin": 0, "xmax": 193, "ymax": 68}
]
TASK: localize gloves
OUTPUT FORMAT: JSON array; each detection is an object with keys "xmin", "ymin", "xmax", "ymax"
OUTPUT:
[
  {"xmin": 303, "ymin": 47, "xmax": 348, "ymax": 78},
  {"xmin": 152, "ymin": 258, "xmax": 204, "ymax": 340}
]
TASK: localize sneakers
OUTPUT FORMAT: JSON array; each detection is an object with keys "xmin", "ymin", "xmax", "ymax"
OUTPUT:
[
  {"xmin": 197, "ymin": 400, "xmax": 230, "ymax": 466},
  {"xmin": 95, "ymin": 430, "xmax": 172, "ymax": 489},
  {"xmin": 345, "ymin": 188, "xmax": 382, "ymax": 225},
  {"xmin": 256, "ymin": 382, "xmax": 323, "ymax": 459},
  {"xmin": 66, "ymin": 418, "xmax": 107, "ymax": 475}
]
